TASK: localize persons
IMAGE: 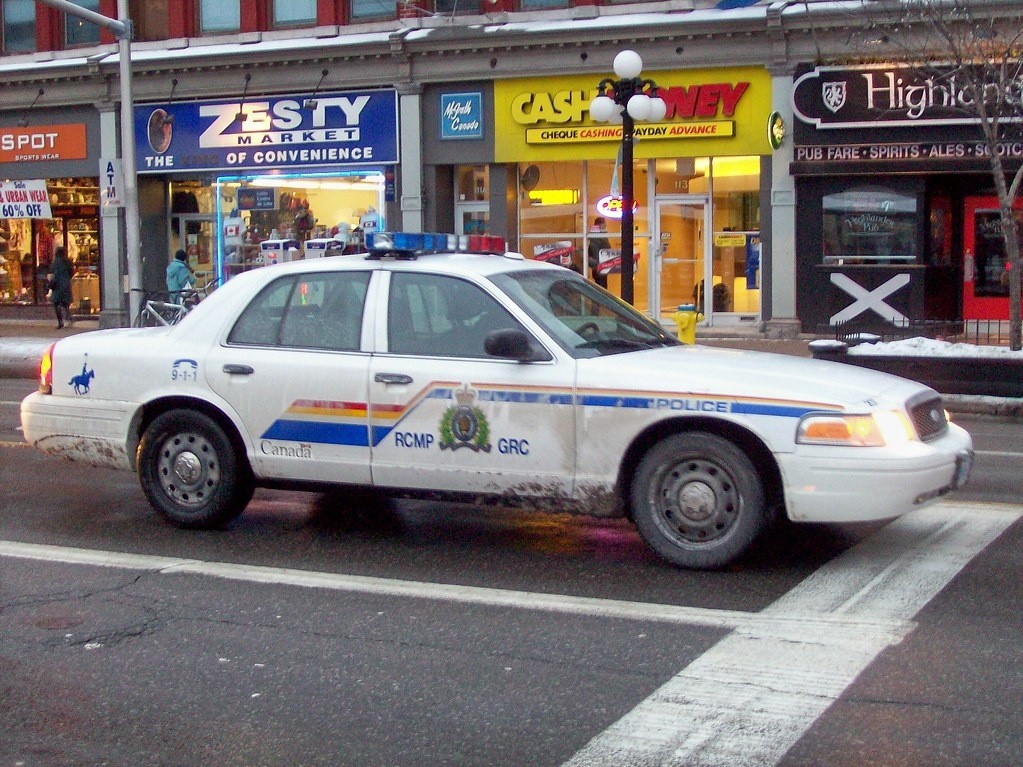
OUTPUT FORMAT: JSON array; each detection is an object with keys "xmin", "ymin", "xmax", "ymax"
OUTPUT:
[
  {"xmin": 358, "ymin": 207, "xmax": 385, "ymax": 245},
  {"xmin": 166, "ymin": 249, "xmax": 200, "ymax": 312},
  {"xmin": 50, "ymin": 247, "xmax": 74, "ymax": 330},
  {"xmin": 588, "ymin": 217, "xmax": 611, "ymax": 316},
  {"xmin": 35, "ymin": 219, "xmax": 78, "ymax": 303}
]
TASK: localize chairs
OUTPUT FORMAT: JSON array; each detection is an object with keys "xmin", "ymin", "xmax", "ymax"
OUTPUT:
[
  {"xmin": 320, "ymin": 282, "xmax": 353, "ymax": 351},
  {"xmin": 437, "ymin": 289, "xmax": 485, "ymax": 359}
]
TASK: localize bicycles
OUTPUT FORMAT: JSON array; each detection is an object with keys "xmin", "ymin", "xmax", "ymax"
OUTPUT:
[{"xmin": 127, "ymin": 278, "xmax": 223, "ymax": 332}]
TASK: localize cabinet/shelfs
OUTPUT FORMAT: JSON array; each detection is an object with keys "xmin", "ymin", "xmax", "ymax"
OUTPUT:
[{"xmin": 33, "ymin": 177, "xmax": 100, "ymax": 267}]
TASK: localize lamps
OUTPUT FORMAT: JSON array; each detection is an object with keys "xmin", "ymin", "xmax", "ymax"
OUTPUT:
[
  {"xmin": 161, "ymin": 78, "xmax": 178, "ymax": 123},
  {"xmin": 234, "ymin": 72, "xmax": 252, "ymax": 121},
  {"xmin": 17, "ymin": 89, "xmax": 44, "ymax": 128},
  {"xmin": 303, "ymin": 68, "xmax": 329, "ymax": 108}
]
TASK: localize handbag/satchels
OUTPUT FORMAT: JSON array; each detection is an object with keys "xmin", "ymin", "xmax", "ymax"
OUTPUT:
[{"xmin": 180, "ymin": 281, "xmax": 195, "ymax": 306}]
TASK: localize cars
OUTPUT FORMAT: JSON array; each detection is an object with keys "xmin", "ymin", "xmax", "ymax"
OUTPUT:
[{"xmin": 19, "ymin": 230, "xmax": 979, "ymax": 571}]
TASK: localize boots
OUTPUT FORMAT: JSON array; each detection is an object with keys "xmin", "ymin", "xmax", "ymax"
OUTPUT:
[
  {"xmin": 65, "ymin": 306, "xmax": 72, "ymax": 320},
  {"xmin": 56, "ymin": 310, "xmax": 64, "ymax": 329}
]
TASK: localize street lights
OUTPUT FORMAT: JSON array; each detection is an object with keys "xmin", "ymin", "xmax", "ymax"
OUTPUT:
[{"xmin": 587, "ymin": 48, "xmax": 670, "ymax": 307}]
